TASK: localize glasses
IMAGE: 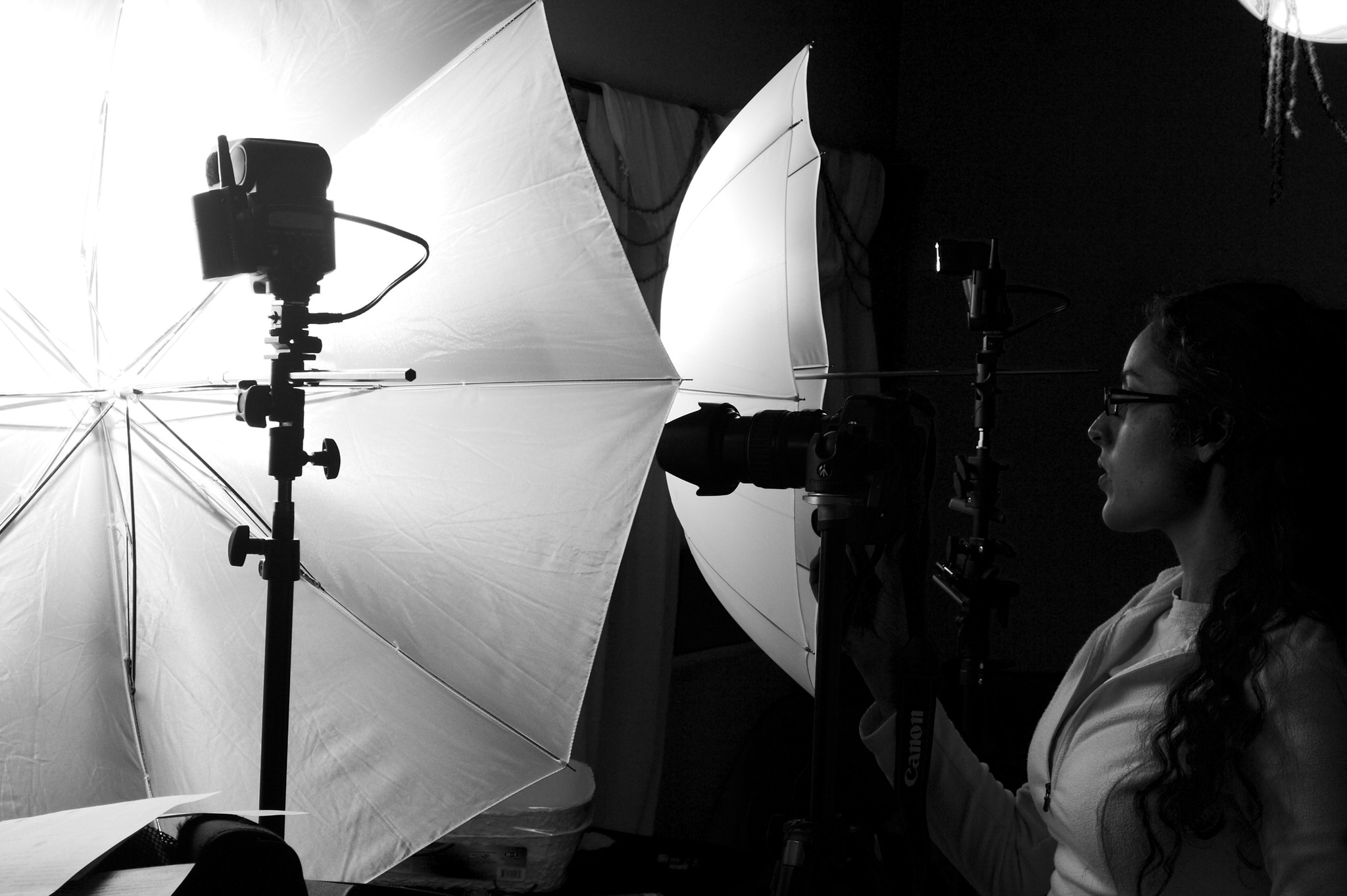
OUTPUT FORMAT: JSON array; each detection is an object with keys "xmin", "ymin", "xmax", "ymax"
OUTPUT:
[{"xmin": 1103, "ymin": 388, "xmax": 1205, "ymax": 416}]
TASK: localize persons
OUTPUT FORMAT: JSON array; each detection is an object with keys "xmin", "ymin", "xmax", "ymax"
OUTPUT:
[{"xmin": 807, "ymin": 280, "xmax": 1347, "ymax": 896}]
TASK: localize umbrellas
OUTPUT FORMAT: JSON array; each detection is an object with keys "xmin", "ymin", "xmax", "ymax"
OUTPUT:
[
  {"xmin": 647, "ymin": 42, "xmax": 841, "ymax": 699},
  {"xmin": 0, "ymin": 0, "xmax": 679, "ymax": 895}
]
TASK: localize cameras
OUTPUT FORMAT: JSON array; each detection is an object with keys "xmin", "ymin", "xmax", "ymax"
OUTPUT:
[
  {"xmin": 660, "ymin": 385, "xmax": 937, "ymax": 496},
  {"xmin": 192, "ymin": 136, "xmax": 336, "ymax": 280}
]
